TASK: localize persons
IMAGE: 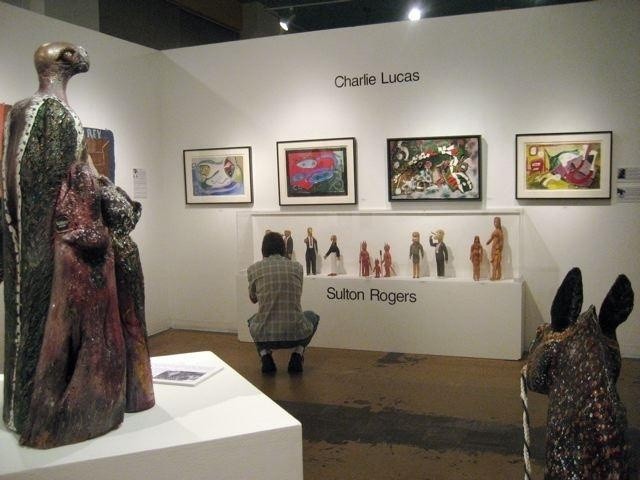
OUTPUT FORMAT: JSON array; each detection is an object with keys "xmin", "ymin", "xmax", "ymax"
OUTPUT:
[
  {"xmin": 373, "ymin": 259, "xmax": 382, "ymax": 278},
  {"xmin": 430, "ymin": 230, "xmax": 448, "ymax": 279},
  {"xmin": 245, "ymin": 232, "xmax": 320, "ymax": 377},
  {"xmin": 359, "ymin": 241, "xmax": 371, "ymax": 276},
  {"xmin": 323, "ymin": 234, "xmax": 340, "ymax": 276},
  {"xmin": 408, "ymin": 231, "xmax": 424, "ymax": 279},
  {"xmin": 381, "ymin": 244, "xmax": 392, "ymax": 277},
  {"xmin": 468, "ymin": 235, "xmax": 483, "ymax": 281},
  {"xmin": 283, "ymin": 229, "xmax": 294, "ymax": 260},
  {"xmin": 304, "ymin": 227, "xmax": 318, "ymax": 276},
  {"xmin": 486, "ymin": 216, "xmax": 504, "ymax": 281}
]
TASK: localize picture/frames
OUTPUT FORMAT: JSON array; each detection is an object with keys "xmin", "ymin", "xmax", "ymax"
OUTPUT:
[
  {"xmin": 387, "ymin": 135, "xmax": 482, "ymax": 201},
  {"xmin": 276, "ymin": 136, "xmax": 358, "ymax": 207},
  {"xmin": 515, "ymin": 131, "xmax": 613, "ymax": 199},
  {"xmin": 183, "ymin": 146, "xmax": 252, "ymax": 204}
]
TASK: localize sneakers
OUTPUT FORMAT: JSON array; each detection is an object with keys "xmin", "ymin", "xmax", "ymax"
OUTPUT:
[
  {"xmin": 288, "ymin": 352, "xmax": 304, "ymax": 372},
  {"xmin": 262, "ymin": 353, "xmax": 276, "ymax": 372}
]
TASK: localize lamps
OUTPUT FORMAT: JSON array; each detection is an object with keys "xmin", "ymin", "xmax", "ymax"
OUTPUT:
[{"xmin": 279, "ymin": 15, "xmax": 297, "ymax": 31}]
